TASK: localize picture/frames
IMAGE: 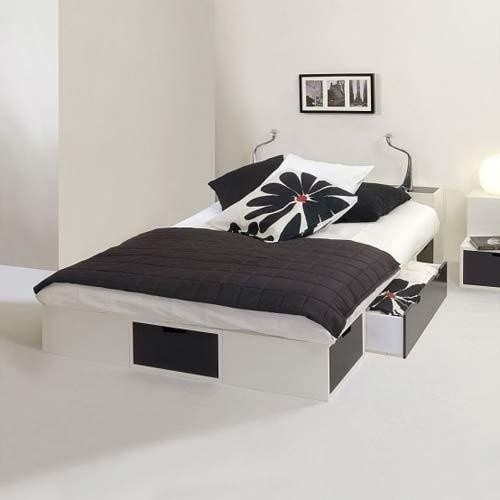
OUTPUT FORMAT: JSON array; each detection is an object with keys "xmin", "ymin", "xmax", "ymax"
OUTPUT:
[{"xmin": 298, "ymin": 74, "xmax": 376, "ymax": 113}]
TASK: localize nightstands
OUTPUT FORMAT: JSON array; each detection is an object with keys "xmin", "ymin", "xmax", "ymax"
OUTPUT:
[{"xmin": 460, "ymin": 190, "xmax": 500, "ymax": 290}]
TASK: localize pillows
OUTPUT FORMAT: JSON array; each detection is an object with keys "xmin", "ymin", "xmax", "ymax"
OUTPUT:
[
  {"xmin": 257, "ymin": 154, "xmax": 376, "ymax": 194},
  {"xmin": 334, "ymin": 182, "xmax": 411, "ymax": 223},
  {"xmin": 208, "ymin": 155, "xmax": 284, "ymax": 210},
  {"xmin": 204, "ymin": 165, "xmax": 358, "ymax": 243}
]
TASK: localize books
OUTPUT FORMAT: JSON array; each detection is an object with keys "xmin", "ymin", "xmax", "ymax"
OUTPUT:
[{"xmin": 469, "ymin": 235, "xmax": 500, "ymax": 251}]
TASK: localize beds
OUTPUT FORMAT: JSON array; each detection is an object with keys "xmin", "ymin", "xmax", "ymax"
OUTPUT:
[{"xmin": 31, "ymin": 127, "xmax": 446, "ymax": 403}]
